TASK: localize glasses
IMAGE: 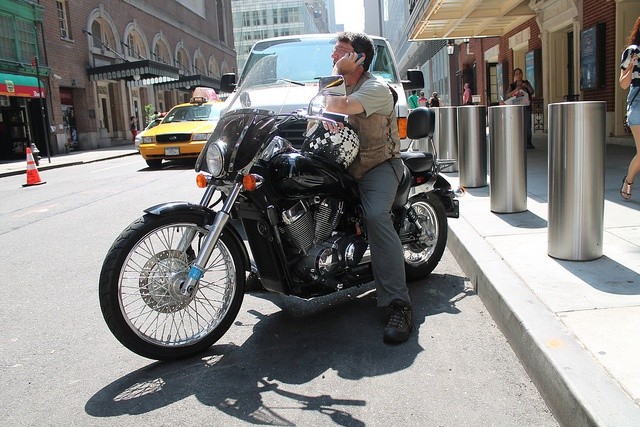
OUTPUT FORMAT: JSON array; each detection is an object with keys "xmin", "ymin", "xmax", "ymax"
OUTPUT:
[
  {"xmin": 463, "ymin": 39, "xmax": 469, "ymax": 43},
  {"xmin": 447, "ymin": 40, "xmax": 454, "ymax": 55}
]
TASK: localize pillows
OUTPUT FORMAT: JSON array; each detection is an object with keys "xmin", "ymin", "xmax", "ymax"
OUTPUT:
[{"xmin": 301, "ymin": 121, "xmax": 360, "ymax": 171}]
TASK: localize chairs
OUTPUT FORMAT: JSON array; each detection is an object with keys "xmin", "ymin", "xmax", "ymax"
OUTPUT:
[
  {"xmin": 382, "ymin": 298, "xmax": 416, "ymax": 344},
  {"xmin": 527, "ymin": 143, "xmax": 535, "ymax": 150},
  {"xmin": 244, "ymin": 271, "xmax": 271, "ymax": 293}
]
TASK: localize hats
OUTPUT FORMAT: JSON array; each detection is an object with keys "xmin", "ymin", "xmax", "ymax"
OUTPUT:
[
  {"xmin": 139, "ymin": 96, "xmax": 225, "ymax": 167},
  {"xmin": 134, "ymin": 116, "xmax": 174, "ymax": 153}
]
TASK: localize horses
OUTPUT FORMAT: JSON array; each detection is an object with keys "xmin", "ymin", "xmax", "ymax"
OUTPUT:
[{"xmin": 356, "ymin": 54, "xmax": 364, "ymax": 64}]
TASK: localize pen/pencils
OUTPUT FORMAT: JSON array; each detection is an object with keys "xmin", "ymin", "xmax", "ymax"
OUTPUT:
[{"xmin": 221, "ymin": 33, "xmax": 425, "ymax": 150}]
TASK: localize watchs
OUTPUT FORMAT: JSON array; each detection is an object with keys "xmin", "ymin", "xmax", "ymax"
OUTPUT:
[{"xmin": 99, "ymin": 76, "xmax": 465, "ymax": 359}]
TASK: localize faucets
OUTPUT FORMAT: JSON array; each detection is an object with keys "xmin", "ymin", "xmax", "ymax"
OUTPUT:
[{"xmin": 620, "ymin": 175, "xmax": 634, "ymax": 200}]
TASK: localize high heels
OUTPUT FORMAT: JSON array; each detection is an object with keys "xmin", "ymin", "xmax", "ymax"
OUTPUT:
[{"xmin": 22, "ymin": 147, "xmax": 47, "ymax": 186}]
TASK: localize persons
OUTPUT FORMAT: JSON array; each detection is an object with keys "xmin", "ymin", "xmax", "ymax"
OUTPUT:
[
  {"xmin": 619, "ymin": 18, "xmax": 640, "ymax": 201},
  {"xmin": 507, "ymin": 68, "xmax": 535, "ymax": 150},
  {"xmin": 72, "ymin": 127, "xmax": 77, "ymax": 140},
  {"xmin": 151, "ymin": 111, "xmax": 168, "ymax": 120},
  {"xmin": 407, "ymin": 89, "xmax": 420, "ymax": 109},
  {"xmin": 417, "ymin": 91, "xmax": 429, "ymax": 107},
  {"xmin": 463, "ymin": 82, "xmax": 471, "ymax": 105},
  {"xmin": 305, "ymin": 31, "xmax": 415, "ymax": 346},
  {"xmin": 130, "ymin": 116, "xmax": 138, "ymax": 142},
  {"xmin": 429, "ymin": 92, "xmax": 439, "ymax": 107}
]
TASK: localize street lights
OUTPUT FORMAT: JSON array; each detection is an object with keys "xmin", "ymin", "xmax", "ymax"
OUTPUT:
[{"xmin": 623, "ymin": 113, "xmax": 632, "ymax": 136}]
